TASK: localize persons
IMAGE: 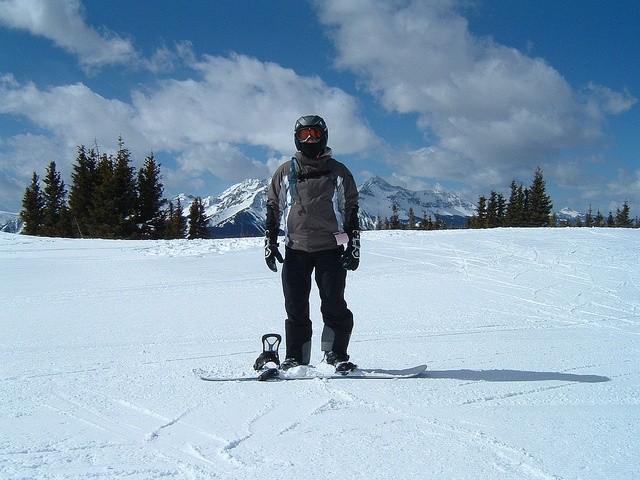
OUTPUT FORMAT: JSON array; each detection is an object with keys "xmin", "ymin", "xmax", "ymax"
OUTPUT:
[{"xmin": 264, "ymin": 115, "xmax": 361, "ymax": 375}]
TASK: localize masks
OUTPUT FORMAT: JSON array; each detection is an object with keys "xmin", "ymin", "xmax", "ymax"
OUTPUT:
[{"xmin": 300, "ymin": 143, "xmax": 322, "ymax": 157}]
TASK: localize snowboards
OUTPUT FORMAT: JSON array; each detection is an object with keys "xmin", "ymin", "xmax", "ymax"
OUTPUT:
[{"xmin": 192, "ymin": 334, "xmax": 427, "ymax": 381}]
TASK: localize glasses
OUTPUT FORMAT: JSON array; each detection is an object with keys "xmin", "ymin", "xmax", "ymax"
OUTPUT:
[{"xmin": 296, "ymin": 127, "xmax": 325, "ymax": 143}]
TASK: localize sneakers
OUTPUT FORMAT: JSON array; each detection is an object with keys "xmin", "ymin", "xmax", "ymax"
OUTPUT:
[
  {"xmin": 279, "ymin": 357, "xmax": 300, "ymax": 370},
  {"xmin": 324, "ymin": 349, "xmax": 355, "ymax": 374}
]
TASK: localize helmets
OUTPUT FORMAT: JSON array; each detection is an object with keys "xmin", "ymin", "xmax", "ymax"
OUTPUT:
[{"xmin": 294, "ymin": 114, "xmax": 328, "ymax": 151}]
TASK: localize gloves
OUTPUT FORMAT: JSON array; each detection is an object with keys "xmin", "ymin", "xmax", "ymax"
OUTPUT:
[
  {"xmin": 339, "ymin": 229, "xmax": 361, "ymax": 271},
  {"xmin": 265, "ymin": 228, "xmax": 284, "ymax": 272}
]
